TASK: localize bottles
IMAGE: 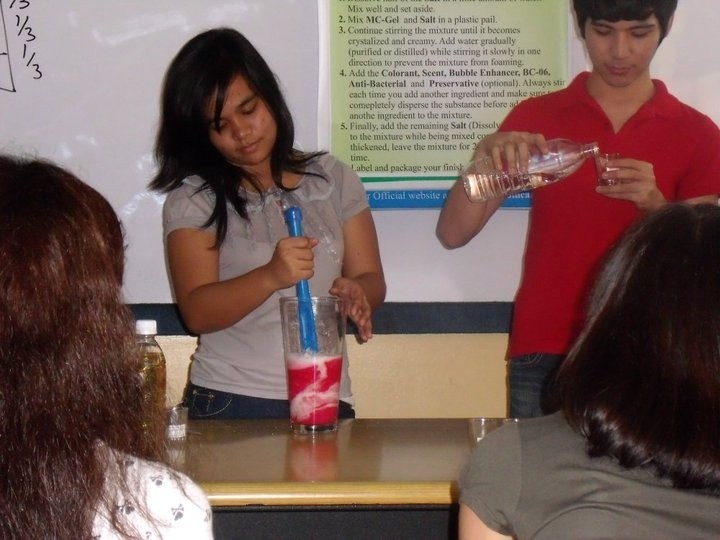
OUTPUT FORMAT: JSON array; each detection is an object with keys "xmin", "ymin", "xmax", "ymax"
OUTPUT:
[
  {"xmin": 461, "ymin": 138, "xmax": 600, "ymax": 204},
  {"xmin": 133, "ymin": 319, "xmax": 167, "ymax": 458}
]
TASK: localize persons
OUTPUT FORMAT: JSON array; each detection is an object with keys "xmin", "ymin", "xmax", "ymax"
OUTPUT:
[
  {"xmin": 0, "ymin": 154, "xmax": 213, "ymax": 539},
  {"xmin": 435, "ymin": 0, "xmax": 720, "ymax": 420},
  {"xmin": 143, "ymin": 28, "xmax": 387, "ymax": 421},
  {"xmin": 456, "ymin": 201, "xmax": 719, "ymax": 540}
]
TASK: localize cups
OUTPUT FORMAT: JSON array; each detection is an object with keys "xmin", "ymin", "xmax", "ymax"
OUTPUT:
[
  {"xmin": 469, "ymin": 418, "xmax": 497, "ymax": 450},
  {"xmin": 595, "ymin": 153, "xmax": 622, "ymax": 187},
  {"xmin": 278, "ymin": 296, "xmax": 350, "ymax": 436},
  {"xmin": 165, "ymin": 407, "xmax": 188, "ymax": 442},
  {"xmin": 503, "ymin": 418, "xmax": 528, "ymax": 425}
]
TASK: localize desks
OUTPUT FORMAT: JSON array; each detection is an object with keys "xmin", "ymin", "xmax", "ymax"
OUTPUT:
[{"xmin": 160, "ymin": 417, "xmax": 503, "ymax": 540}]
